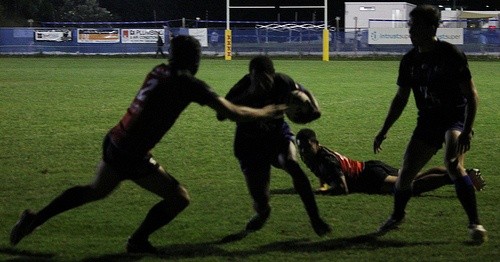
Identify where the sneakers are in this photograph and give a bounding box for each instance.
[465,224,488,243]
[474,169,486,186]
[11,208,37,249]
[378,212,405,236]
[466,169,482,190]
[125,238,167,257]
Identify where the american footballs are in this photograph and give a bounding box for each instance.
[284,91,310,124]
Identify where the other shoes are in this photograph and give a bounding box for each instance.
[246,207,270,233]
[313,219,334,236]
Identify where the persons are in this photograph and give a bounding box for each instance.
[154,32,166,59]
[478,32,487,57]
[218,56,331,237]
[8,34,289,259]
[372,4,488,241]
[295,129,487,197]
